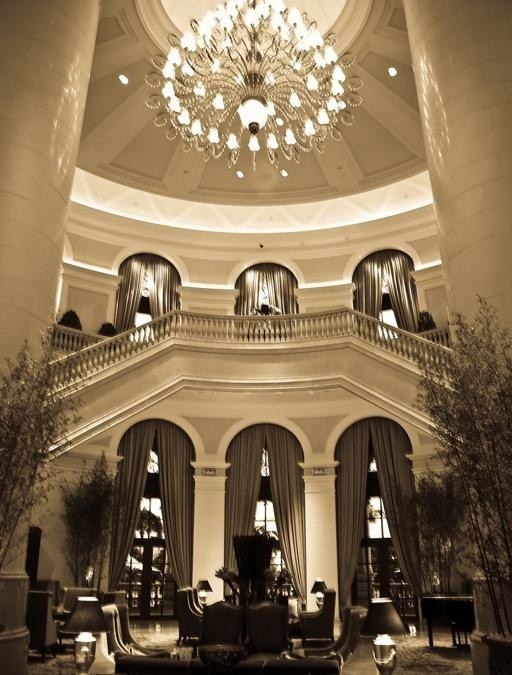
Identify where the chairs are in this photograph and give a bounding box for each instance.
[26,574,474,675]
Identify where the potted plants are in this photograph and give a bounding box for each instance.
[408,297,512,675]
[408,460,474,653]
[1,306,95,675]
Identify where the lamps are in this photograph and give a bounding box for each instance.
[141,0,365,184]
